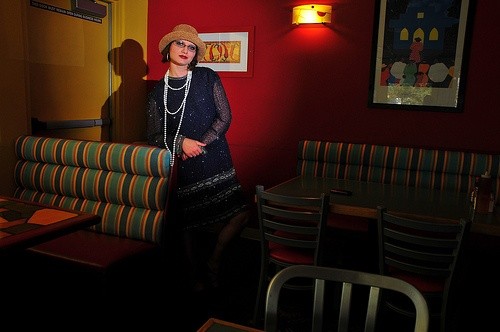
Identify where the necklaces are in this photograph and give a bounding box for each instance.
[163,67,192,166]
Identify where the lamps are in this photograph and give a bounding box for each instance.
[293,4,332,24]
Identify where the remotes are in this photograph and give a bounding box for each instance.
[328,188,352,196]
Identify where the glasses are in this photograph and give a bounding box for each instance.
[173,40,198,52]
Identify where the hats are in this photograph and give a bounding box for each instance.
[159,24,205,62]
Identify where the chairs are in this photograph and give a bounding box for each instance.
[253,185,472,332]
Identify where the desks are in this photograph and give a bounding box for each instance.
[255,174,500,247]
[0,195,101,252]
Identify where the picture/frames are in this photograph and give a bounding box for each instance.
[367,0,477,113]
[194,24,256,78]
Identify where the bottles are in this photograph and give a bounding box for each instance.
[475,171,492,215]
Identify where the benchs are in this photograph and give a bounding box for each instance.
[12,136,174,280]
[284,138,500,273]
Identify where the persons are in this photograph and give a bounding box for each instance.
[144,24,251,292]
